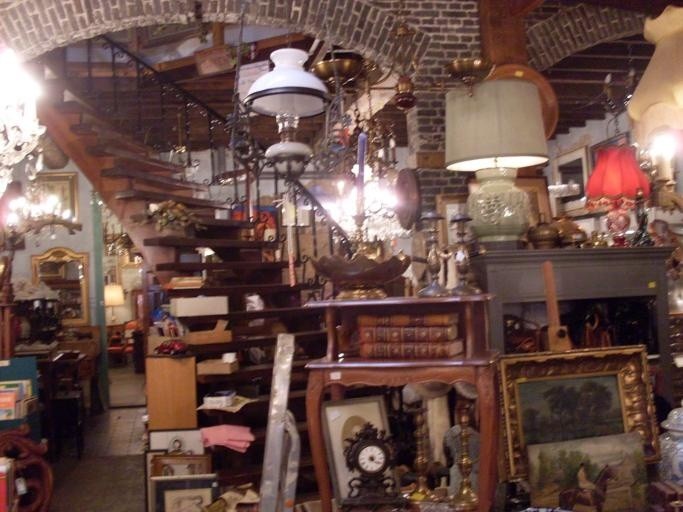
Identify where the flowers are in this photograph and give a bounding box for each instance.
[130,199,208,233]
[127,320,145,333]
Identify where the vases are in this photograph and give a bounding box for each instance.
[132,331,145,374]
[159,222,188,239]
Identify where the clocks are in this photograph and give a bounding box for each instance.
[339,423,409,512]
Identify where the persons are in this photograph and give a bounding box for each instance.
[576,462,603,500]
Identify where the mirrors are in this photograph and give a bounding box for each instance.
[30,248,90,328]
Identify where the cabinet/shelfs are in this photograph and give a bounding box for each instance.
[304,296,501,512]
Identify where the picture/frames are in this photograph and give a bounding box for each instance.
[70,27,138,49]
[144,429,219,512]
[104,233,122,284]
[320,395,399,508]
[119,247,144,268]
[130,289,144,321]
[137,22,213,50]
[29,172,78,227]
[501,345,663,483]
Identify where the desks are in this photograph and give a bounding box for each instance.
[105,321,128,367]
[473,245,676,410]
[34,350,102,463]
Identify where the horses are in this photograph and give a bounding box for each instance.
[559,463,620,512]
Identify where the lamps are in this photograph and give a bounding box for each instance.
[103,282,122,322]
[241,1,683,302]
